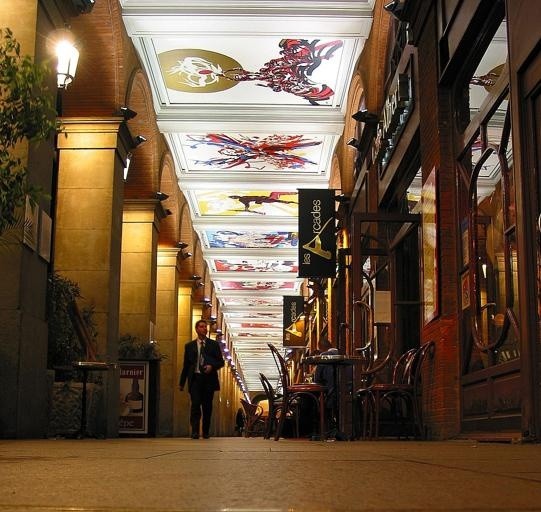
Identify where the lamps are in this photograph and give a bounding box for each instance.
[56,39,81,89]
[133,135,147,147]
[192,272,249,396]
[185,252,192,257]
[154,191,169,201]
[166,208,172,216]
[175,241,189,249]
[121,106,137,121]
[336,108,371,204]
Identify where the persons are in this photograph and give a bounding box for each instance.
[178,320,224,440]
[233,408,244,434]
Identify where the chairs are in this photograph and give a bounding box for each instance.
[241,342,434,440]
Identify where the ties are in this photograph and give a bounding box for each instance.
[199,342,206,372]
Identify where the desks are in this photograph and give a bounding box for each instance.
[51,359,119,439]
